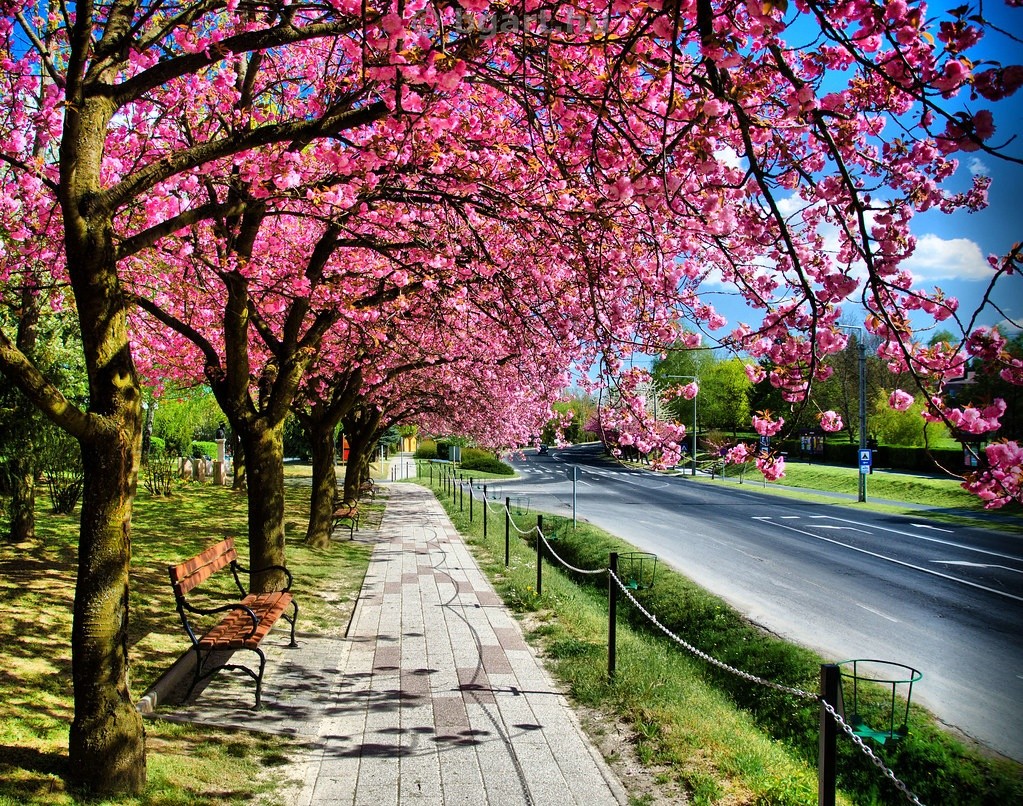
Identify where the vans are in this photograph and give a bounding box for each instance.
[538,444,549,456]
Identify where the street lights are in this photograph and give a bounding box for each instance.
[834,321,866,503]
[667,374,697,476]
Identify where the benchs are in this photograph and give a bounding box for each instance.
[332,496,360,540]
[167,534,299,710]
[359,476,376,500]
